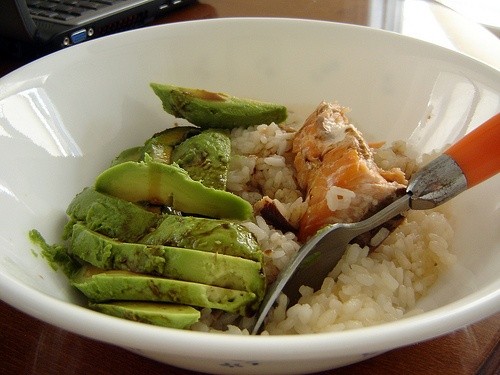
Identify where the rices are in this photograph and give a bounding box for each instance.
[185,102,457,337]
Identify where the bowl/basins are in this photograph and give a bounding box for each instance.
[0,16,500,375]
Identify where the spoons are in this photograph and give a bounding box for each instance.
[254,116,500,335]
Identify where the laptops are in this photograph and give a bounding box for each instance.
[1,0,200,53]
